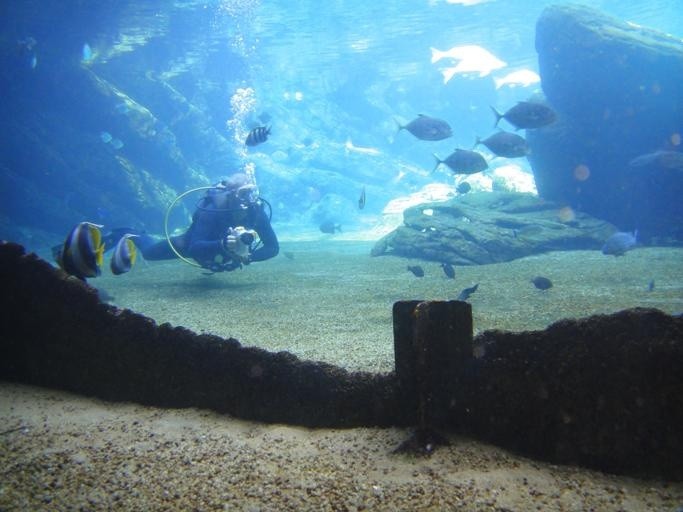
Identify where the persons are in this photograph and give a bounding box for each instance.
[51,172,278,281]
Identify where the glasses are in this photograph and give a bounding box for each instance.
[237,184,259,202]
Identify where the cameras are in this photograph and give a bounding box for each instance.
[233,227,258,257]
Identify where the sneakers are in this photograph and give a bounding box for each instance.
[102,232,123,249]
